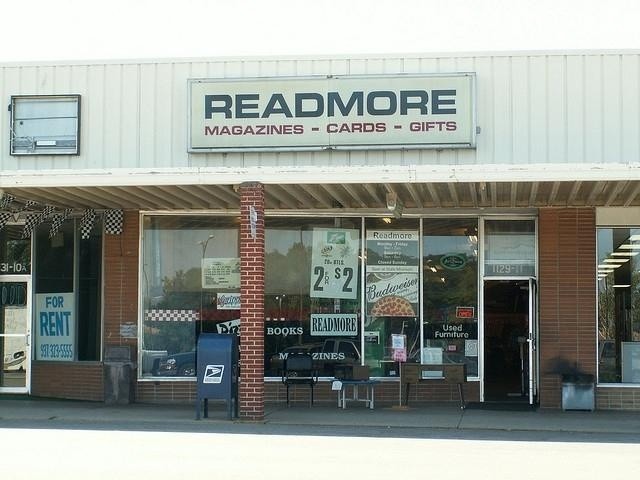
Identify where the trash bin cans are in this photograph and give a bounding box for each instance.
[562,374,595,413]
[102,346,136,405]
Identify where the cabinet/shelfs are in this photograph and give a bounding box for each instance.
[401,361,468,411]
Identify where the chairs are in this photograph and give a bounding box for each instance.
[282,350,320,407]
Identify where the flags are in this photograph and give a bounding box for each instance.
[0,194,16,209]
[0,212,12,231]
[104,209,123,235]
[41,205,55,223]
[62,208,73,219]
[80,214,96,240]
[79,208,95,229]
[21,200,37,212]
[20,214,42,240]
[49,214,65,241]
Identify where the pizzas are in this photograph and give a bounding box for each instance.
[371,295,416,315]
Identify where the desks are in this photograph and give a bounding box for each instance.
[333,380,382,411]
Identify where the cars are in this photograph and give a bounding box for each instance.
[152,352,195,377]
[270,338,361,368]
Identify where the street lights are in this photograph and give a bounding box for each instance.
[275,294,286,313]
[198,235,215,333]
